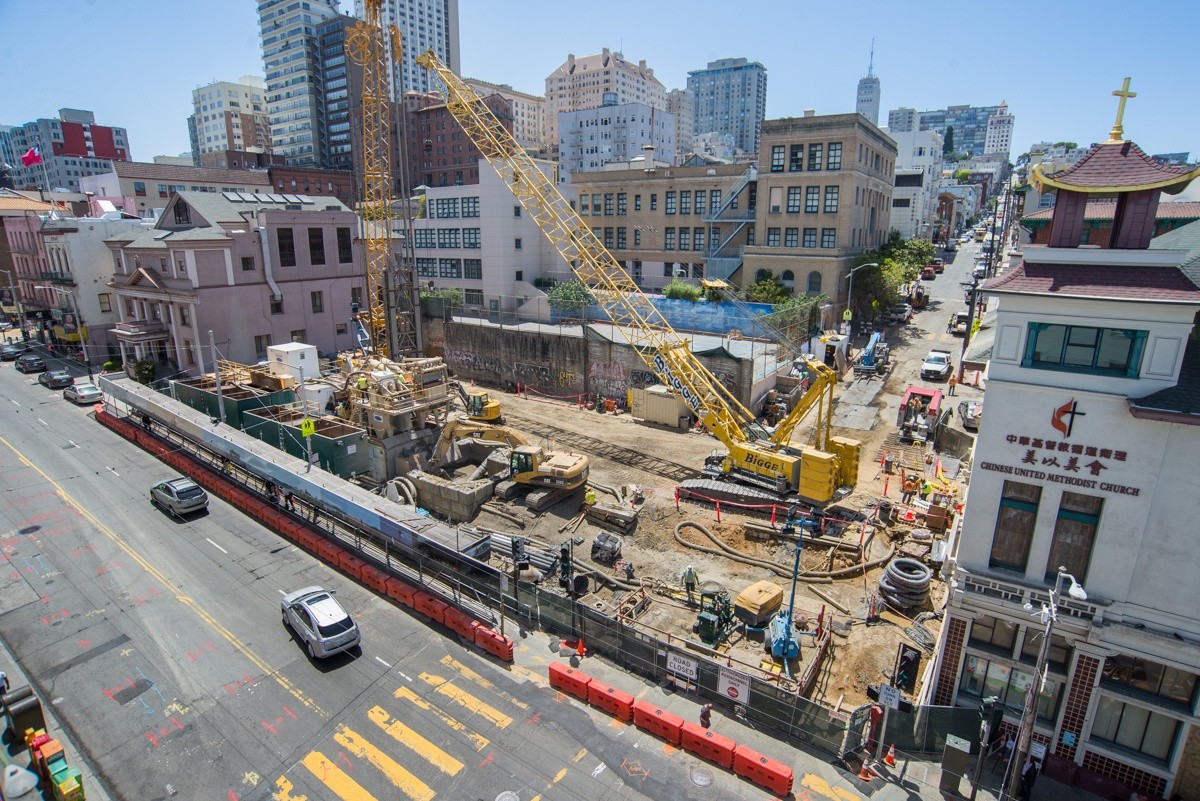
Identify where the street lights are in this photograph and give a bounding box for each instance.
[34,285,95,384]
[1005,566,1088,800]
[910,223,930,239]
[258,359,314,465]
[945,199,961,228]
[845,263,879,326]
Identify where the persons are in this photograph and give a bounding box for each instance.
[62,314,76,331]
[909,395,922,423]
[325,371,405,424]
[48,344,85,364]
[699,703,713,729]
[948,375,957,395]
[681,566,699,601]
[902,475,916,505]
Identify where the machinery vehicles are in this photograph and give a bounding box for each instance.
[973,220,992,242]
[415,47,862,513]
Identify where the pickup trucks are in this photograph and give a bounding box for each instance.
[889,302,913,322]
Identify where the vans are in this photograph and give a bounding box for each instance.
[982,218,1003,250]
[965,282,981,304]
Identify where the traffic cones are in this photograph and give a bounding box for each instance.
[884,743,897,766]
[859,758,872,781]
[574,639,586,656]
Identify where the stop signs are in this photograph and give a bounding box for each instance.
[727,687,739,698]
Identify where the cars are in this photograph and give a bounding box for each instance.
[973,260,988,278]
[952,312,970,333]
[0,344,104,405]
[280,585,361,661]
[946,224,982,252]
[150,477,209,518]
[921,257,945,279]
[920,349,952,379]
[957,398,983,429]
